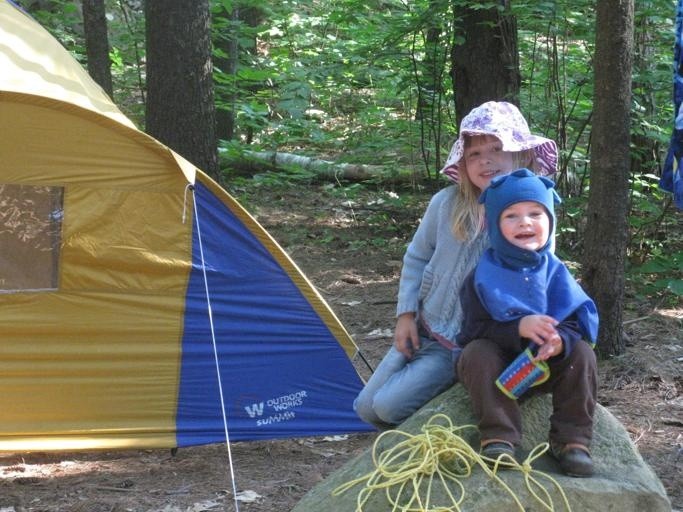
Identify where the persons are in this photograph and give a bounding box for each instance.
[454,167,601,480]
[351,99,559,434]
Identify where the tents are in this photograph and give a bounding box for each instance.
[0,0,377,456]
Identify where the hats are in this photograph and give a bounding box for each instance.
[435,98,559,192]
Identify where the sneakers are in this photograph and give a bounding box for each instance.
[477,441,518,471]
[546,435,595,480]
